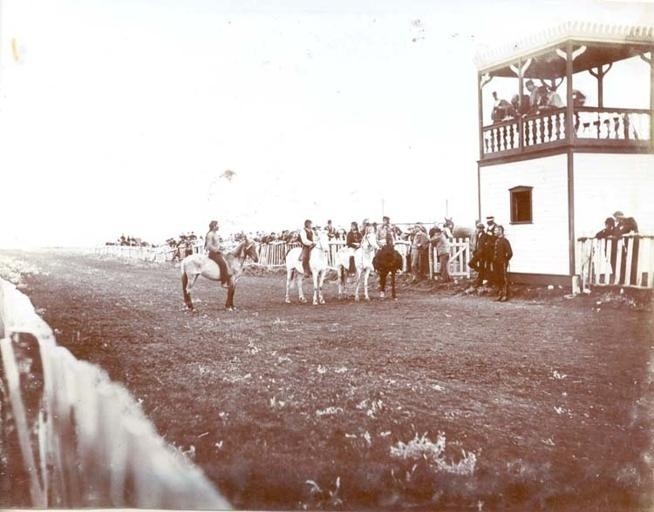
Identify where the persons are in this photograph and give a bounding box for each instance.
[247,221,402,242]
[300,220,318,278]
[205,222,229,290]
[490,77,587,145]
[167,231,198,262]
[611,211,641,284]
[219,232,246,242]
[377,217,395,248]
[347,223,363,278]
[595,216,622,276]
[402,223,453,283]
[465,216,515,302]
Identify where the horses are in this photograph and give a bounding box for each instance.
[180,235,258,313]
[372,230,403,301]
[284,229,330,305]
[335,229,379,302]
